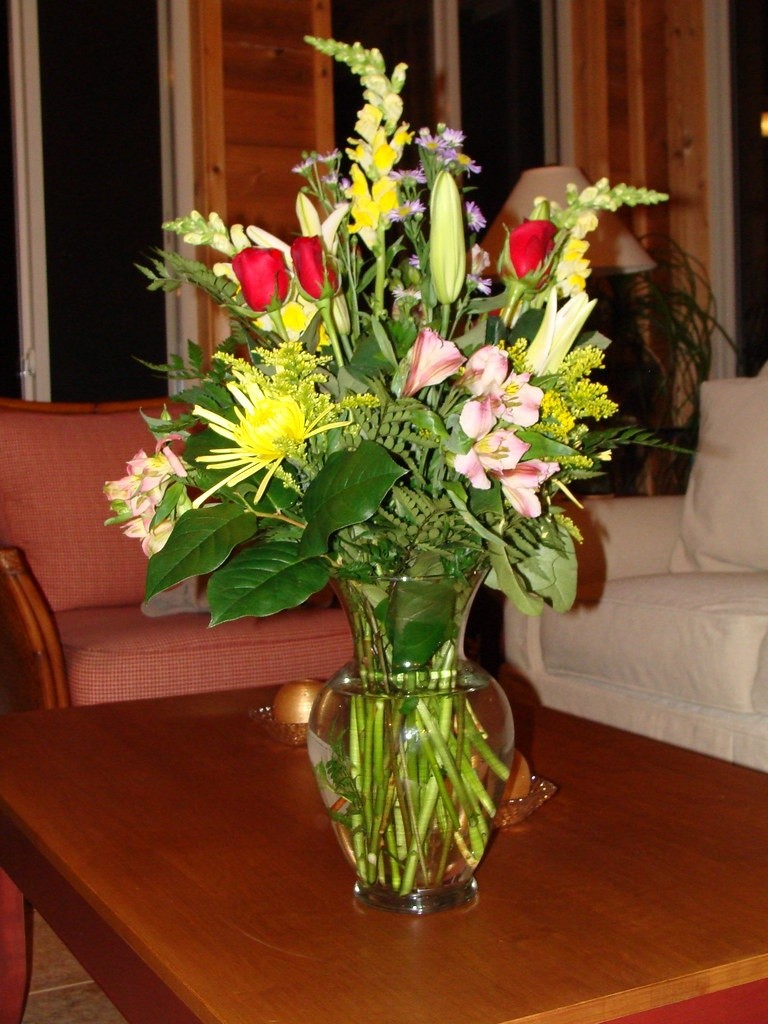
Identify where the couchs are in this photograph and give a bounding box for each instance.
[504,360,768,771]
[0,399,356,1024]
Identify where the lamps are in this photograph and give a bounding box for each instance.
[464,164,658,281]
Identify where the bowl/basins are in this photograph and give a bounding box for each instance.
[488,777,556,829]
[248,706,308,746]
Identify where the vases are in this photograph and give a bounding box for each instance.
[307,559,517,917]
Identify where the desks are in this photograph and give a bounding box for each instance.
[0,680,768,1024]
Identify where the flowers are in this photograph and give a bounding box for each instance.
[100,34,671,628]
